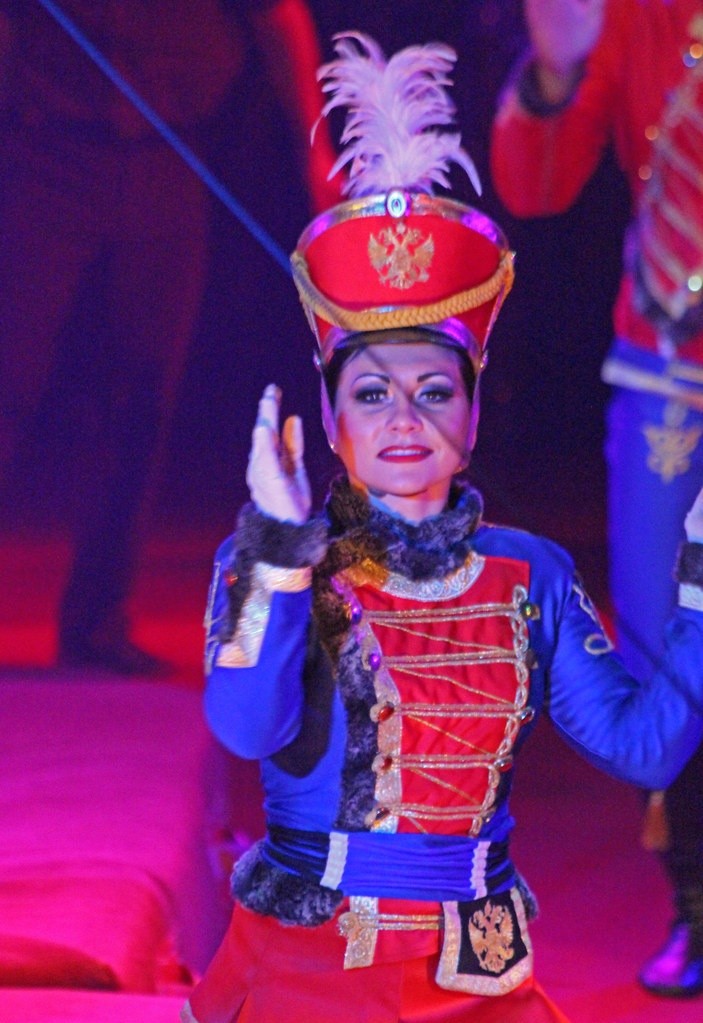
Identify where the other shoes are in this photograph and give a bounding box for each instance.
[635,887,702,997]
[63,634,169,679]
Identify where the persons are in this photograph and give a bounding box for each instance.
[180,187,703,1022]
[1,0,352,681]
[482,0,702,995]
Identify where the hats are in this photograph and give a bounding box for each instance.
[290,32,515,371]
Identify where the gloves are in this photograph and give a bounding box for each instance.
[522,1,603,72]
[244,383,311,523]
